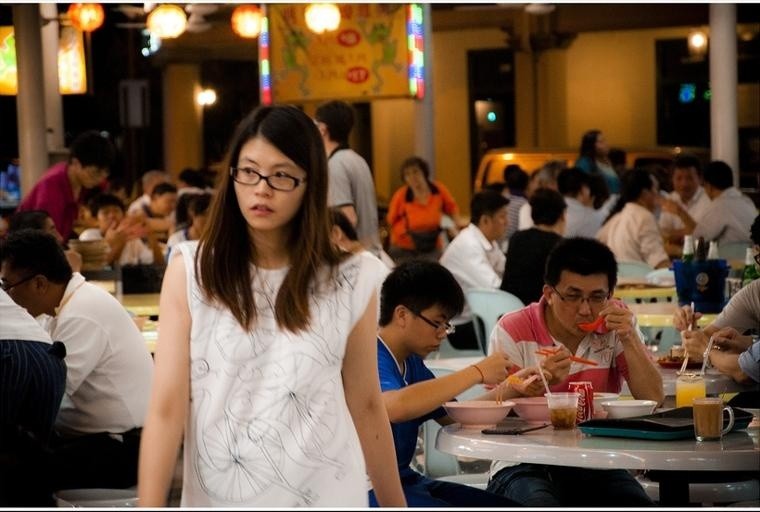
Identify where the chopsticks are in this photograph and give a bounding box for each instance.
[490,335,505,406]
[701,336,714,372]
[535,347,600,368]
[680,352,690,374]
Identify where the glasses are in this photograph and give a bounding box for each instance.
[401,304,457,335]
[551,285,611,306]
[228,166,308,192]
[0,276,35,292]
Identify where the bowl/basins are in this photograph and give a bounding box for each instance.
[67,238,110,269]
[442,390,658,430]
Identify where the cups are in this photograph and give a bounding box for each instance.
[675,376,706,411]
[691,438,727,471]
[690,396,735,441]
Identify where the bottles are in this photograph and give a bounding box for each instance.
[741,245,759,291]
[681,234,721,263]
[723,269,741,310]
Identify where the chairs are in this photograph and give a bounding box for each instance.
[631,472,759,509]
[613,286,679,305]
[712,240,753,262]
[617,259,654,282]
[462,287,527,361]
[433,310,484,357]
[421,364,497,493]
[621,302,720,328]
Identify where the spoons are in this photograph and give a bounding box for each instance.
[575,314,606,332]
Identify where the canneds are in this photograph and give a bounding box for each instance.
[567,381,593,423]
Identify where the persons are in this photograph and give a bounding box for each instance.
[0,103,759,508]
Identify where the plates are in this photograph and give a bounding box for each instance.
[657,357,704,371]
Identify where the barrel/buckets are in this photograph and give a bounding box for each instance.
[668,259,731,314]
